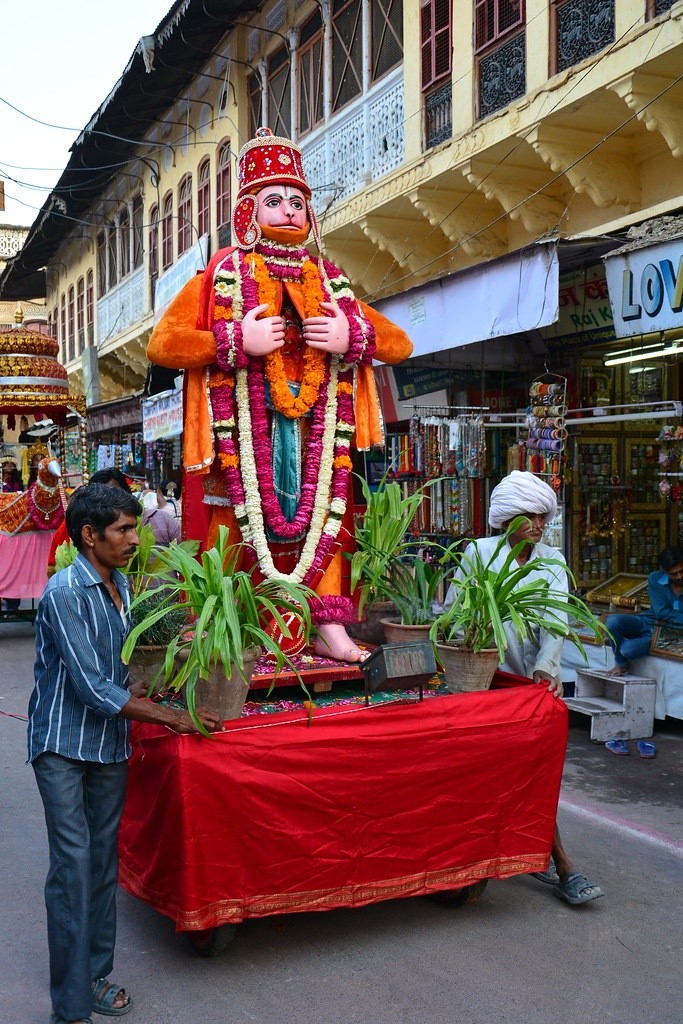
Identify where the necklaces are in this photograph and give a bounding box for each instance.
[203,243,361,606]
[390,413,485,539]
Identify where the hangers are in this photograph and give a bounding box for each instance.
[527,361,567,477]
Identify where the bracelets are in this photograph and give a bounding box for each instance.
[524,383,569,451]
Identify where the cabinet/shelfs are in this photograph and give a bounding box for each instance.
[564,357,683,662]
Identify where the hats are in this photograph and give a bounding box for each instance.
[487,469,558,528]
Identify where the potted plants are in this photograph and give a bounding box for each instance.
[342,443,618,692]
[53,513,332,738]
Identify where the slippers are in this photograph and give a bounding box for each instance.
[532,860,560,884]
[89,977,134,1016]
[48,1010,93,1024]
[605,739,629,755]
[553,872,604,904]
[637,741,657,758]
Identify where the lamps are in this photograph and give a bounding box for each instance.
[360,640,438,707]
[601,338,683,368]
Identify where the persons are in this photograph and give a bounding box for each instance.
[0,455,23,494]
[46,469,183,604]
[145,139,414,665]
[605,545,683,676]
[443,470,605,904]
[23,453,45,491]
[26,484,225,1024]
